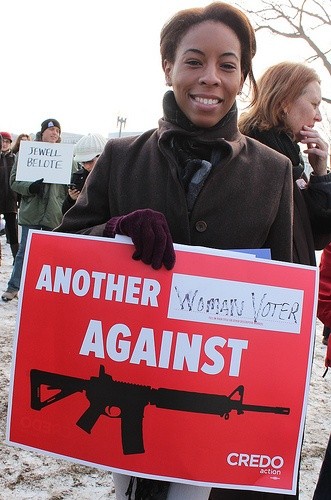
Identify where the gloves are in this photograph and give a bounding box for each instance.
[102,209,176,271]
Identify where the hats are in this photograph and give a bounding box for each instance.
[73,133,107,162]
[0,131,13,143]
[41,119,61,136]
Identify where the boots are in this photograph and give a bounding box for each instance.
[9,243,20,266]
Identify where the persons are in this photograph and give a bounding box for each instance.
[61,134,107,215]
[311,242,331,500]
[239,60,331,251]
[1,119,79,301]
[0,131,20,265]
[11,134,30,153]
[51,0,294,500]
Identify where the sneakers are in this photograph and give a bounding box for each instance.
[2,286,19,301]
